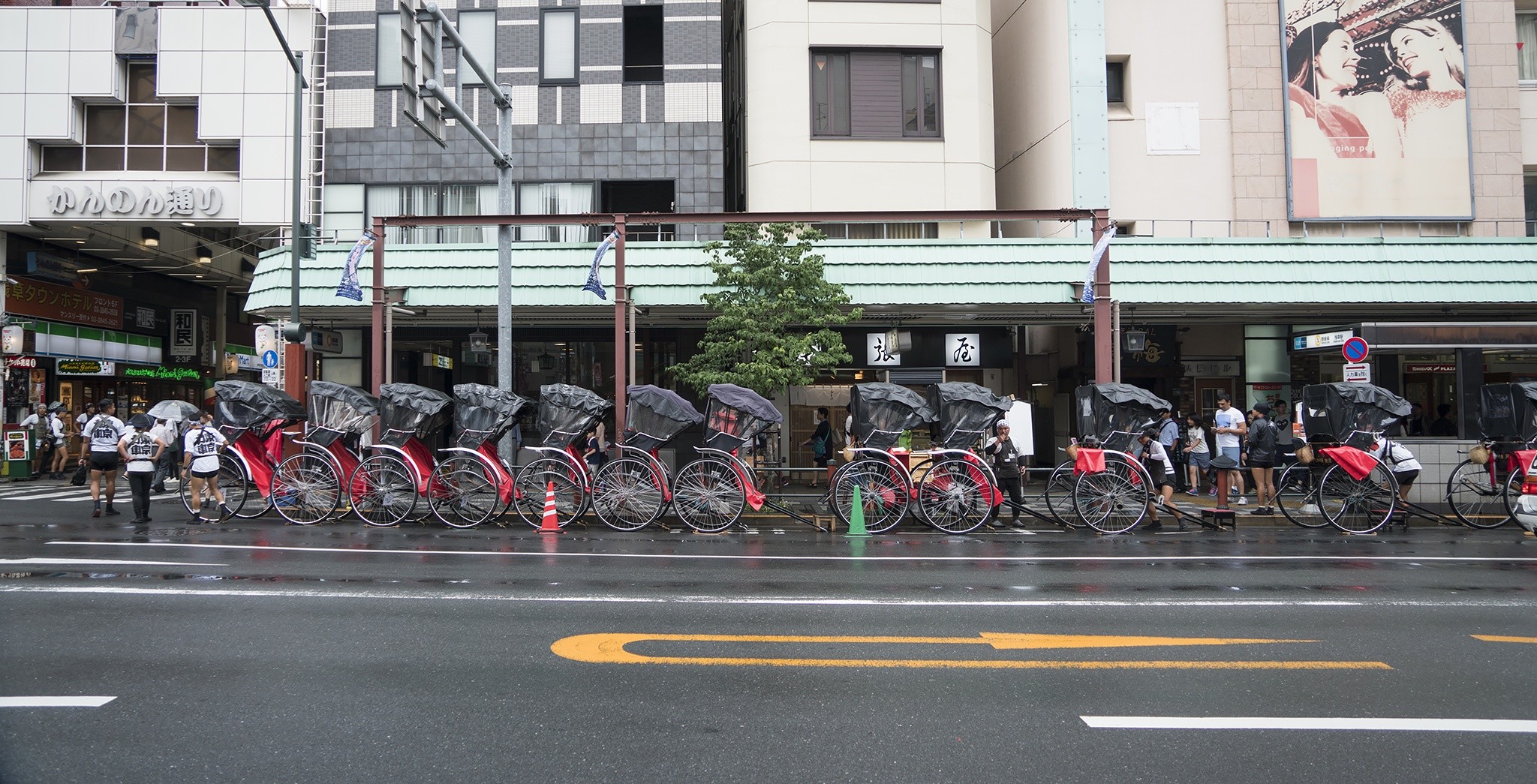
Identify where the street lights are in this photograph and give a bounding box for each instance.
[235,1,310,485]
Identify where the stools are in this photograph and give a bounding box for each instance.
[1367,510,1407,534]
[813,513,837,532]
[1200,509,1235,531]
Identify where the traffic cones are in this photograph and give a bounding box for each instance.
[845,484,874,537]
[537,481,563,534]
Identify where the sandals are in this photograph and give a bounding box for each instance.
[806,482,817,488]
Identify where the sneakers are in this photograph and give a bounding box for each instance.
[1185,484,1303,515]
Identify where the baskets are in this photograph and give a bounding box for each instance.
[1066,443,1083,461]
[1469,445,1490,464]
[1294,444,1314,464]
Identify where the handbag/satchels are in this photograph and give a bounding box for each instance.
[811,441,824,454]
[45,434,57,443]
[71,466,86,486]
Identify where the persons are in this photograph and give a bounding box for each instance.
[1137,429,1188,530]
[20,398,229,524]
[1400,403,1424,437]
[581,418,606,507]
[1182,411,1218,496]
[798,402,890,489]
[1211,393,1248,505]
[1241,399,1307,515]
[338,408,373,508]
[1125,408,1188,499]
[513,421,522,451]
[348,246,362,285]
[1225,433,1245,503]
[985,419,1026,527]
[1287,15,1468,159]
[1429,403,1453,437]
[1367,433,1422,529]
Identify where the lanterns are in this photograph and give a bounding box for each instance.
[226,353,238,374]
[255,324,275,361]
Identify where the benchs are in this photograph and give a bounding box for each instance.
[713,447,739,491]
[563,443,591,488]
[641,445,663,491]
[888,447,919,499]
[307,434,338,490]
[214,421,248,478]
[473,445,491,487]
[390,435,427,487]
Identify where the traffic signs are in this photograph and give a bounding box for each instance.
[1343,362,1371,383]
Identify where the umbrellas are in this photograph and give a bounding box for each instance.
[146,400,200,421]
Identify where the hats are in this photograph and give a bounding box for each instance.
[1253,401,1270,414]
[47,401,64,410]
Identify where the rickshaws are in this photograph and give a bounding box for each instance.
[903,382,1076,536]
[1035,382,1227,535]
[1442,381,1537,537]
[1276,381,1470,534]
[270,380,431,528]
[816,382,996,535]
[589,385,749,533]
[671,383,829,534]
[348,382,515,531]
[174,379,344,525]
[426,382,590,530]
[511,383,672,532]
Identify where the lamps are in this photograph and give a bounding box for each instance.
[468,309,489,354]
[241,257,255,278]
[538,342,554,368]
[1122,306,1147,351]
[197,242,213,263]
[143,225,160,248]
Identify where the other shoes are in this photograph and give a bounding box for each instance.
[1142,523,1163,529]
[143,516,152,521]
[1178,518,1188,529]
[154,485,162,493]
[220,510,229,522]
[1012,519,1026,527]
[774,479,789,488]
[168,477,180,482]
[92,508,100,517]
[203,503,209,507]
[164,478,171,483]
[130,518,142,522]
[50,472,67,481]
[105,507,120,514]
[32,472,43,478]
[186,517,200,524]
[992,520,1006,527]
[121,475,128,480]
